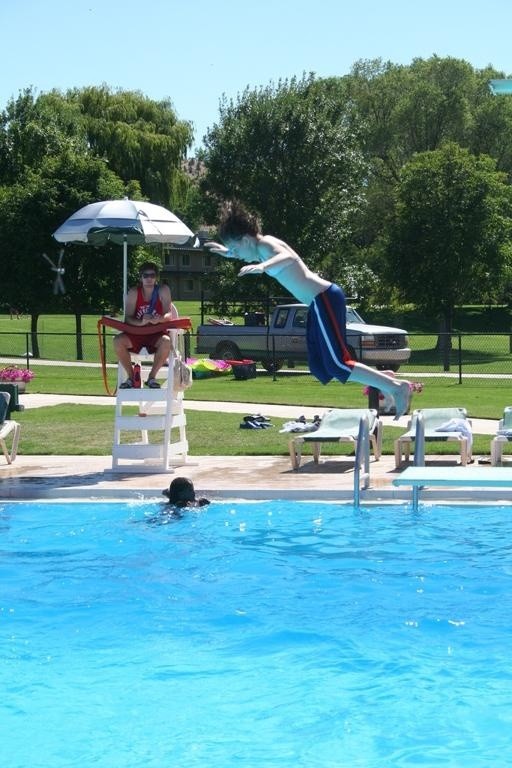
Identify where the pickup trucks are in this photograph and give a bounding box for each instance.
[194,303,412,373]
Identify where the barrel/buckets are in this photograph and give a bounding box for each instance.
[245,312,256,325]
[256,309,265,326]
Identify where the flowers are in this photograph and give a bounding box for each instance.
[0,365,35,385]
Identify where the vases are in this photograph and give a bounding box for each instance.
[1,379,26,394]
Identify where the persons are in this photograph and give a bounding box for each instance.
[204,207,410,422]
[151,478,211,523]
[112,262,173,388]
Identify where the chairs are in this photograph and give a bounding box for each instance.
[286,407,511,474]
[104,303,198,477]
[1,382,25,465]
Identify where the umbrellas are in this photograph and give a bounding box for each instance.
[50,195,200,321]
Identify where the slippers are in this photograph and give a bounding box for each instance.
[119,378,134,389]
[144,378,161,388]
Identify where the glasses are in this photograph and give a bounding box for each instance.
[142,273,156,278]
